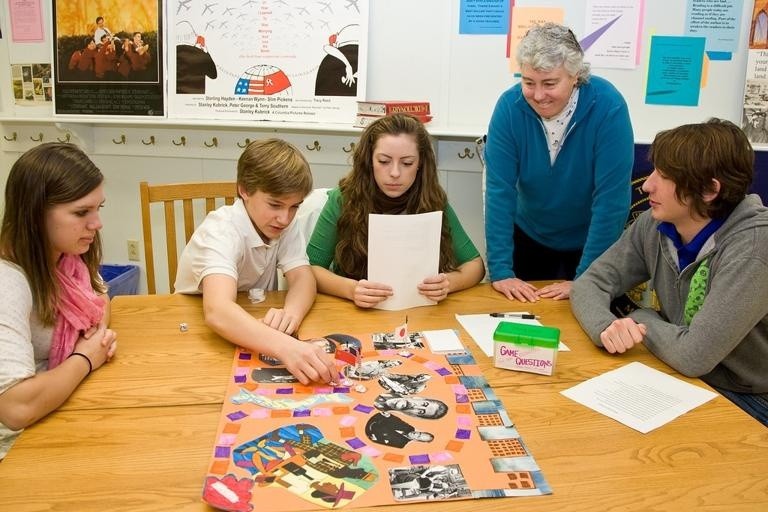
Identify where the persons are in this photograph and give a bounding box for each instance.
[306,114,486,313]
[0,142,119,464]
[174,136,342,387]
[63,15,153,82]
[301,324,464,503]
[481,22,635,303]
[567,119,767,428]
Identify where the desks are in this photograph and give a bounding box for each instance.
[0,280,767,512]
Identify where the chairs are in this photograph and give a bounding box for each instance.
[139,180,237,294]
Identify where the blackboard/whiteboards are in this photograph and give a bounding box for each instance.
[0,0,755,147]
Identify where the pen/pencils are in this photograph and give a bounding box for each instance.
[489,313,541,319]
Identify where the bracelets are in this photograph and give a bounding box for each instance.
[67,353,92,377]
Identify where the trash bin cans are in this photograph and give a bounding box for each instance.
[97,263,140,301]
